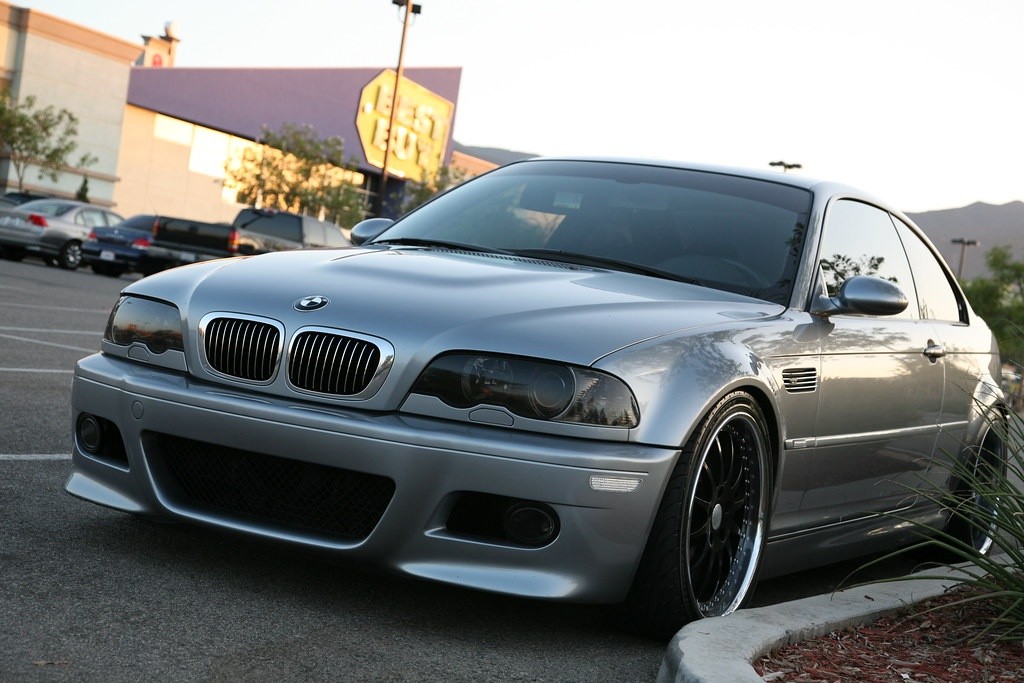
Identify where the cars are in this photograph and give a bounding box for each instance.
[0,190,54,219]
[80,211,162,277]
[0,198,129,271]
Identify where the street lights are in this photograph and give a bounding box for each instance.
[374,0,423,218]
[950,237,982,283]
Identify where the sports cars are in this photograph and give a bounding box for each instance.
[63,157,1016,639]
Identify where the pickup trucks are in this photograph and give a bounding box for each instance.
[139,204,355,280]
